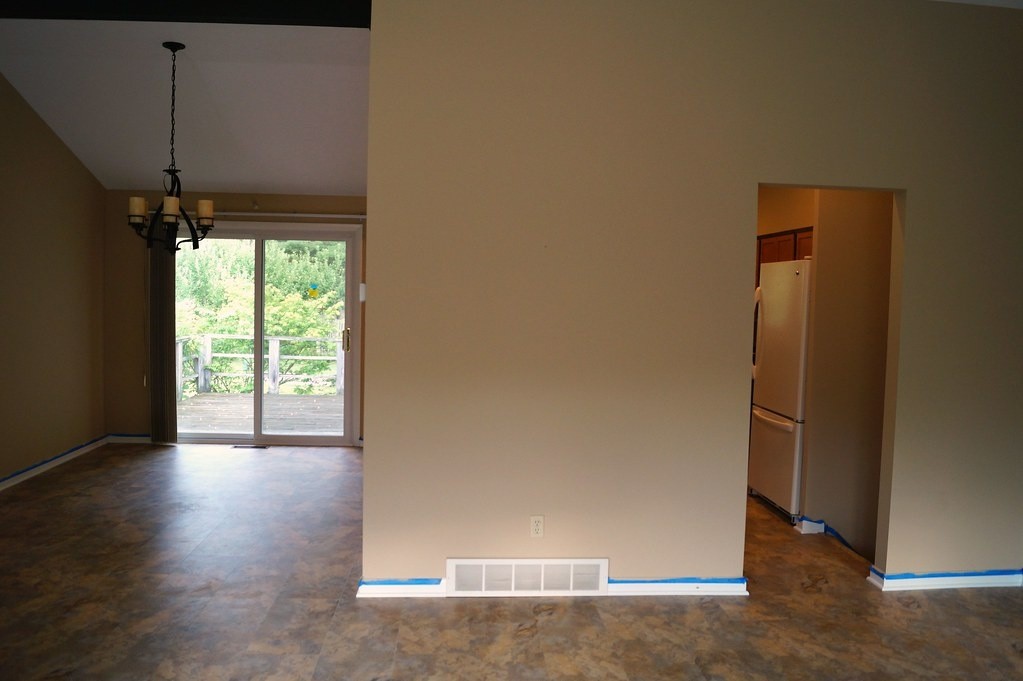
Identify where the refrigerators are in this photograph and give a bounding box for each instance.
[747,255,812,525]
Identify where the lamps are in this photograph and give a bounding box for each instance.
[126,41,215,256]
